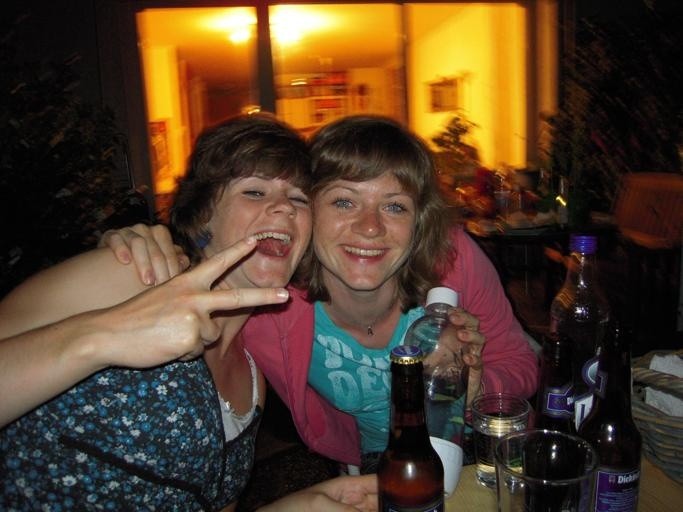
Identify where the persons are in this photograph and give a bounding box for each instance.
[99,116,539,475]
[0,113,378,508]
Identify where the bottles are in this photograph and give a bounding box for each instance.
[574,322,642,511]
[522,334,577,511]
[377,344,445,511]
[403,287,470,498]
[550,235,608,432]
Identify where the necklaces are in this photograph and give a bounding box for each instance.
[335,304,396,336]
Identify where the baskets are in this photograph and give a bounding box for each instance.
[631,351,683,484]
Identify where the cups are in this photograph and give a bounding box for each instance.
[470,393,530,490]
[429,436,464,499]
[448,201,469,227]
[492,427,601,512]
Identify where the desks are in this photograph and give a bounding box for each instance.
[445,463,683,512]
[592,209,682,307]
[462,187,579,305]
